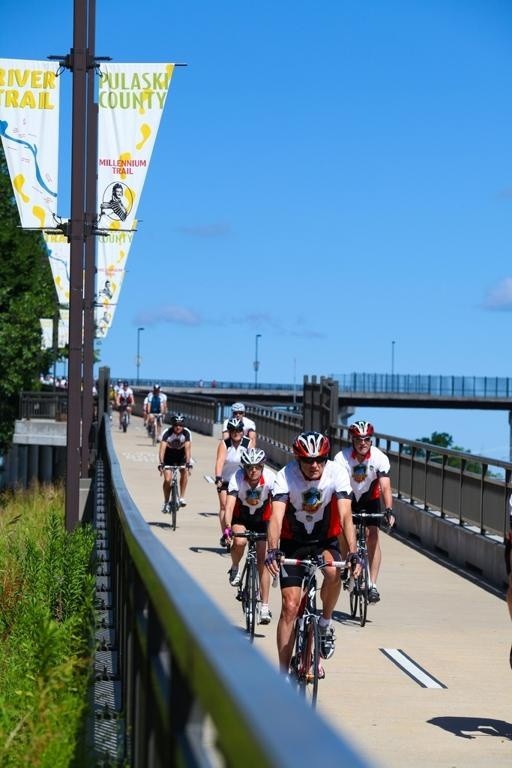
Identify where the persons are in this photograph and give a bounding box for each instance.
[39,374,168,442]
[505,492,512,669]
[216,418,252,546]
[264,432,367,676]
[225,448,276,622]
[222,403,256,448]
[158,413,192,513]
[334,421,395,603]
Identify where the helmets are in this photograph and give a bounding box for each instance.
[154,384,161,389]
[292,430,330,457]
[241,448,266,466]
[348,420,374,439]
[117,379,127,385]
[170,413,186,425]
[227,417,244,428]
[231,403,246,412]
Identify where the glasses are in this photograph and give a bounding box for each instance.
[229,427,244,432]
[298,456,326,463]
[247,463,263,470]
[351,435,370,443]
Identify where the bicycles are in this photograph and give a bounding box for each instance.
[220,529,271,638]
[160,465,191,530]
[341,506,396,627]
[275,551,360,712]
[145,412,166,446]
[116,403,133,433]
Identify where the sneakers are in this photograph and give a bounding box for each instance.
[221,534,226,546]
[341,558,348,580]
[179,498,186,507]
[317,618,335,660]
[369,588,380,603]
[162,503,169,513]
[229,568,241,586]
[260,612,272,622]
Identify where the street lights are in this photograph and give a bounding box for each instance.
[136,327,145,384]
[391,340,397,392]
[252,333,263,388]
